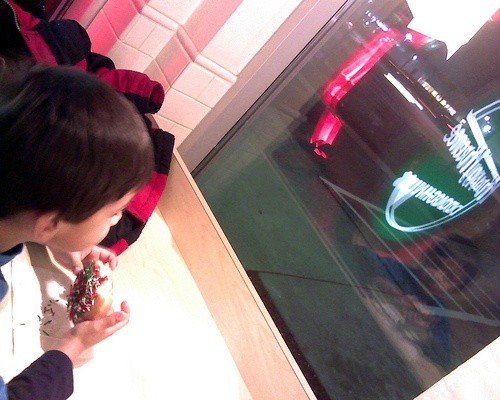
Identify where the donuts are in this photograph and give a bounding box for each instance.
[65,260,114,326]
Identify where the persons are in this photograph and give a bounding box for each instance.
[338,219,487,353]
[0,65,155,400]
